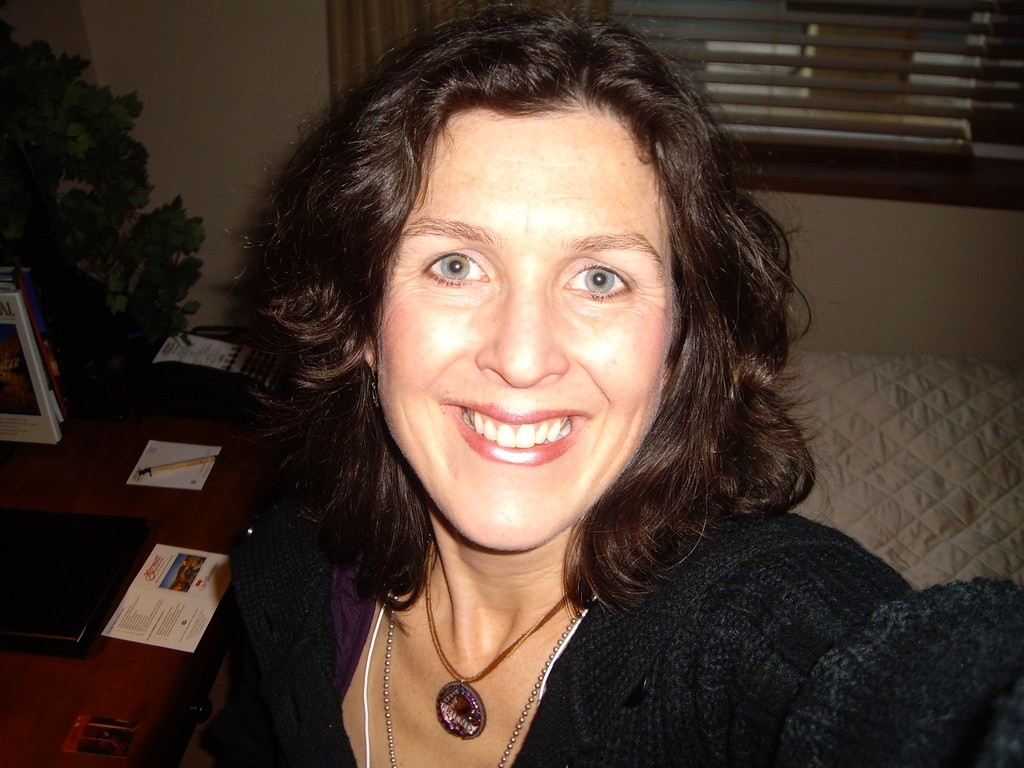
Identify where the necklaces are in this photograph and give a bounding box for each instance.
[427,538,570,741]
[383,593,583,767]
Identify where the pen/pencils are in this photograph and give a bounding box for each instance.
[137,456,219,475]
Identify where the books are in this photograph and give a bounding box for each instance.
[1,506,152,659]
[0,264,69,445]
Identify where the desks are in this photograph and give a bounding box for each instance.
[1,414,279,768]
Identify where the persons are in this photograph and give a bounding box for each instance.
[203,5,1024,767]
